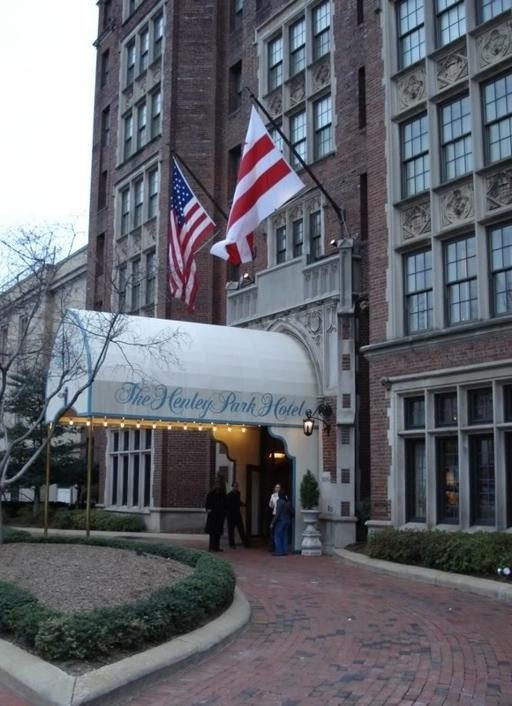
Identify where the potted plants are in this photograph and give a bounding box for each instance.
[300,470,322,557]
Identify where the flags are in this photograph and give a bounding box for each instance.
[210,100,306,267]
[167,154,217,312]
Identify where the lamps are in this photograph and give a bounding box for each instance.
[303,404,332,436]
[330,240,338,253]
[243,272,253,281]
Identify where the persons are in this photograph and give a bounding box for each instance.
[226,481,249,550]
[268,482,288,539]
[204,474,228,553]
[268,490,295,555]
